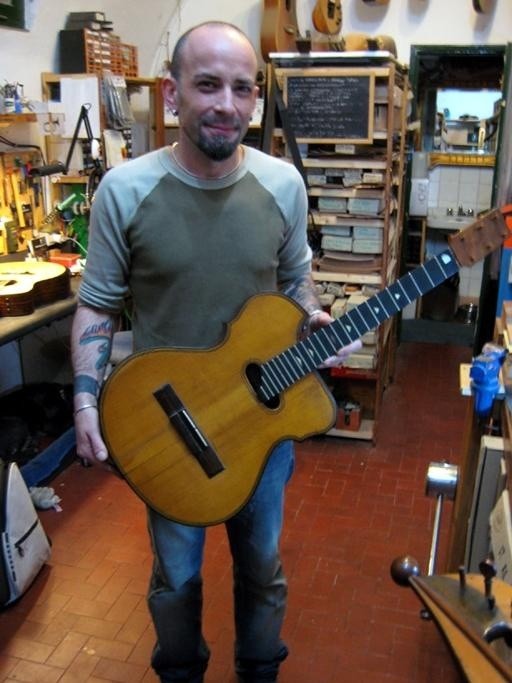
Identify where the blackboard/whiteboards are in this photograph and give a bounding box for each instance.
[282,68,375,145]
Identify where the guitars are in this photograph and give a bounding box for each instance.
[0,262,71,318]
[261,1,300,63]
[96,211,508,526]
[312,1,342,35]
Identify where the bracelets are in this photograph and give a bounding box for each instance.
[73,404,98,417]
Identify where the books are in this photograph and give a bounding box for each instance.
[304,165,397,371]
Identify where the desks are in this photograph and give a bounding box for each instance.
[1,242,83,489]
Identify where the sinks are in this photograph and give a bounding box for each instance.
[427,210,473,229]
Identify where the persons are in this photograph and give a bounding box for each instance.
[70,21,364,682]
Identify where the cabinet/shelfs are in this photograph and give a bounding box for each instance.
[1,110,66,180]
[43,52,410,443]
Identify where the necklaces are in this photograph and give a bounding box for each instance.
[170,141,243,182]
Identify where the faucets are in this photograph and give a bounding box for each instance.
[457,204,465,215]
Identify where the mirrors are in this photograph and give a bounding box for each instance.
[414,84,502,152]
